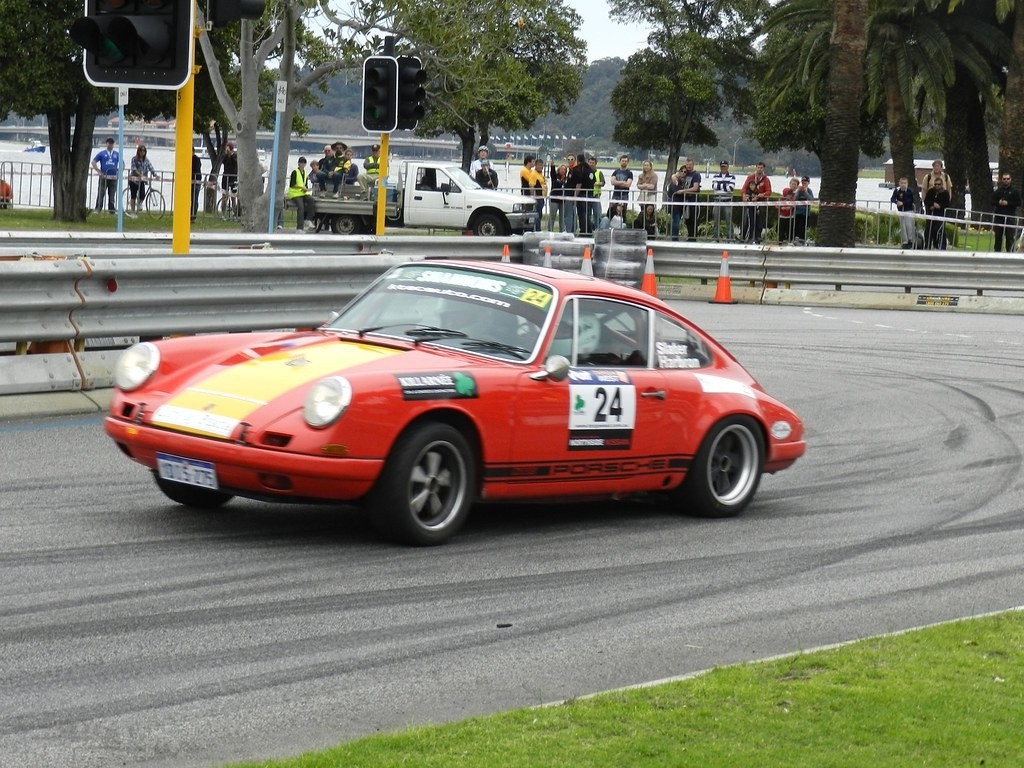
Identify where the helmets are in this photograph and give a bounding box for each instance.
[547,311,601,363]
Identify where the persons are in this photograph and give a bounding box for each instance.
[129,145,160,214]
[992,172,1021,253]
[741,161,814,246]
[191,148,202,222]
[711,161,736,244]
[547,310,602,366]
[669,159,702,241]
[469,145,494,179]
[607,203,626,224]
[476,160,498,190]
[91,138,124,215]
[221,143,238,221]
[890,160,953,250]
[357,144,389,201]
[287,156,316,234]
[638,204,657,239]
[548,153,606,233]
[308,142,359,199]
[637,160,658,210]
[610,155,633,206]
[520,156,548,232]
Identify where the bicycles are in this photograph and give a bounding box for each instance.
[216,181,241,222]
[122,178,166,221]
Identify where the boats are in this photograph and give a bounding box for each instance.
[24,145,46,153]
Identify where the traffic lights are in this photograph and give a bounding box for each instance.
[361,55,399,134]
[395,55,427,132]
[205,0,266,32]
[70,0,194,89]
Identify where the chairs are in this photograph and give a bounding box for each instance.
[588,340,643,366]
[422,176,436,191]
[440,305,521,347]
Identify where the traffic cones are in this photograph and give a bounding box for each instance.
[542,245,552,269]
[579,247,594,277]
[640,248,658,299]
[500,244,511,263]
[709,250,739,305]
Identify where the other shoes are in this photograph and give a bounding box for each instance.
[304,219,315,228]
[277,225,282,229]
[131,214,138,218]
[332,192,338,198]
[138,204,142,211]
[296,229,306,234]
[320,190,327,198]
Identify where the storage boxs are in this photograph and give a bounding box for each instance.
[374,183,397,202]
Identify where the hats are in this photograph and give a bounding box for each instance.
[720,161,729,166]
[371,144,380,150]
[331,141,347,151]
[802,176,810,182]
[478,146,489,154]
[106,138,114,144]
[481,159,490,165]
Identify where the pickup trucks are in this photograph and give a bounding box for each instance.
[311,159,540,236]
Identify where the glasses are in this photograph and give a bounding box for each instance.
[680,170,686,173]
[1003,178,1010,180]
[568,159,573,161]
[934,184,940,186]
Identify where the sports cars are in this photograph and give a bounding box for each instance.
[103,258,807,549]
